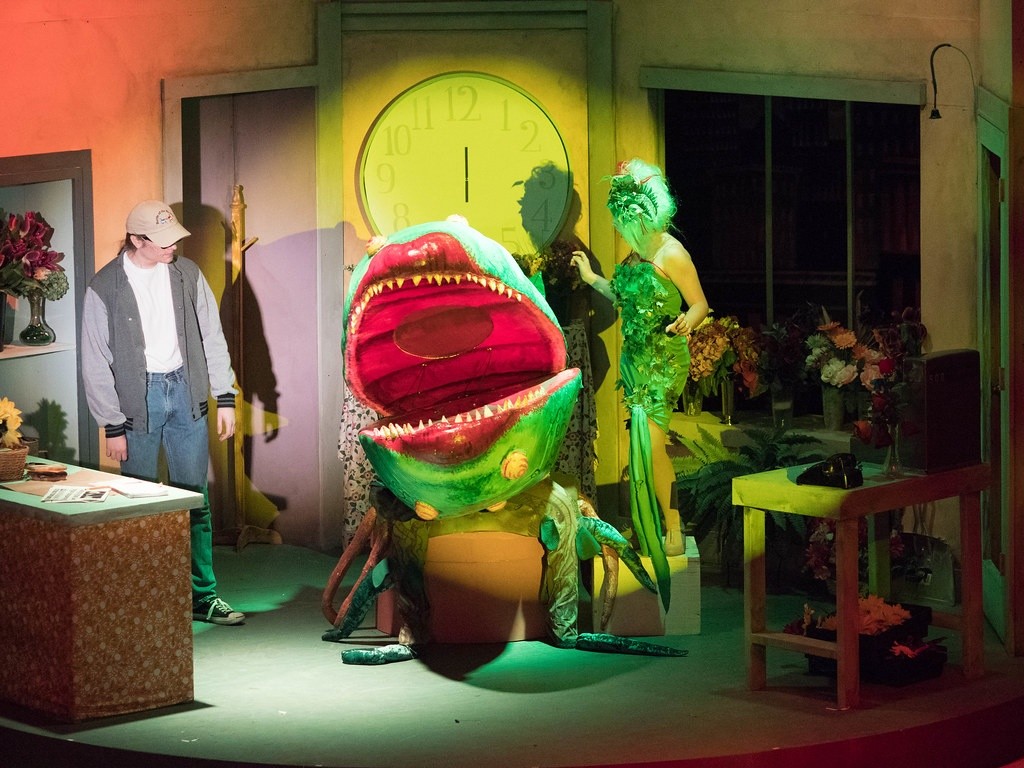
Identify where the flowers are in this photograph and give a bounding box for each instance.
[762,308,807,390]
[783,601,814,635]
[807,319,863,384]
[0,210,70,300]
[694,308,714,330]
[851,322,924,441]
[687,321,762,385]
[0,397,24,449]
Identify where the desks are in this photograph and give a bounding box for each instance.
[730,453,984,712]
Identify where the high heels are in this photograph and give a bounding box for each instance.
[663,526,687,556]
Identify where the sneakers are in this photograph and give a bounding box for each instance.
[192,597,245,624]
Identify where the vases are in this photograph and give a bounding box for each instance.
[0,292,7,352]
[818,387,845,432]
[0,447,29,481]
[684,381,703,417]
[18,297,56,343]
[718,387,737,425]
[881,420,903,478]
[773,381,793,431]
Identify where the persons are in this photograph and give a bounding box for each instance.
[567,159,709,556]
[81,201,244,624]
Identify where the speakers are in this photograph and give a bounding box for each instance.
[901,349,981,473]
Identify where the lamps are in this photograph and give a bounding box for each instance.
[928,41,974,118]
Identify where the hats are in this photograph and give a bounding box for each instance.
[126,200,192,248]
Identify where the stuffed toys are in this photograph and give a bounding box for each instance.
[320,213,690,665]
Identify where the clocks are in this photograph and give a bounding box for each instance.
[354,71,573,260]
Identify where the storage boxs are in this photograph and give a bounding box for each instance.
[593,534,701,635]
[803,600,948,686]
